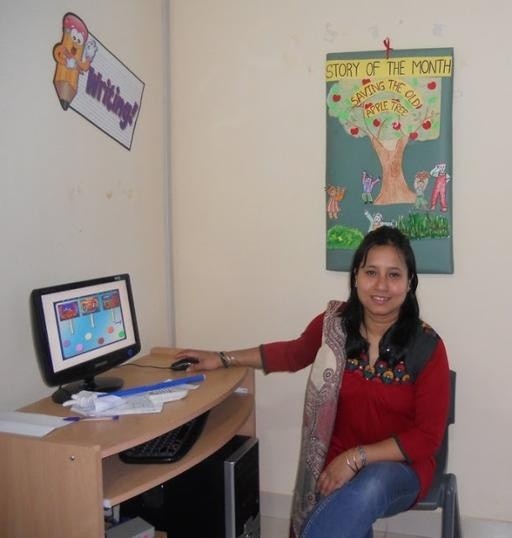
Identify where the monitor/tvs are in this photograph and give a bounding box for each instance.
[29,273,141,404]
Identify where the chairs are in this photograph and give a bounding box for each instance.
[410,370,462,538]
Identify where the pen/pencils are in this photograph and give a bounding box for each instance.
[63,416,119,421]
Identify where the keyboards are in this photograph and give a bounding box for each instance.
[118,409,210,463]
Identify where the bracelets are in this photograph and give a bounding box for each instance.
[358,443,367,467]
[216,350,240,368]
[344,450,358,473]
[350,447,359,472]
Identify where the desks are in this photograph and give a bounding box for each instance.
[0,346,256,538]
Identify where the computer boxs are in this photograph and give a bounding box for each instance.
[118,434,261,537]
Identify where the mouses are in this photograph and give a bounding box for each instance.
[171,358,200,371]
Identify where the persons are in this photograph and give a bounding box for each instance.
[173,223,454,537]
